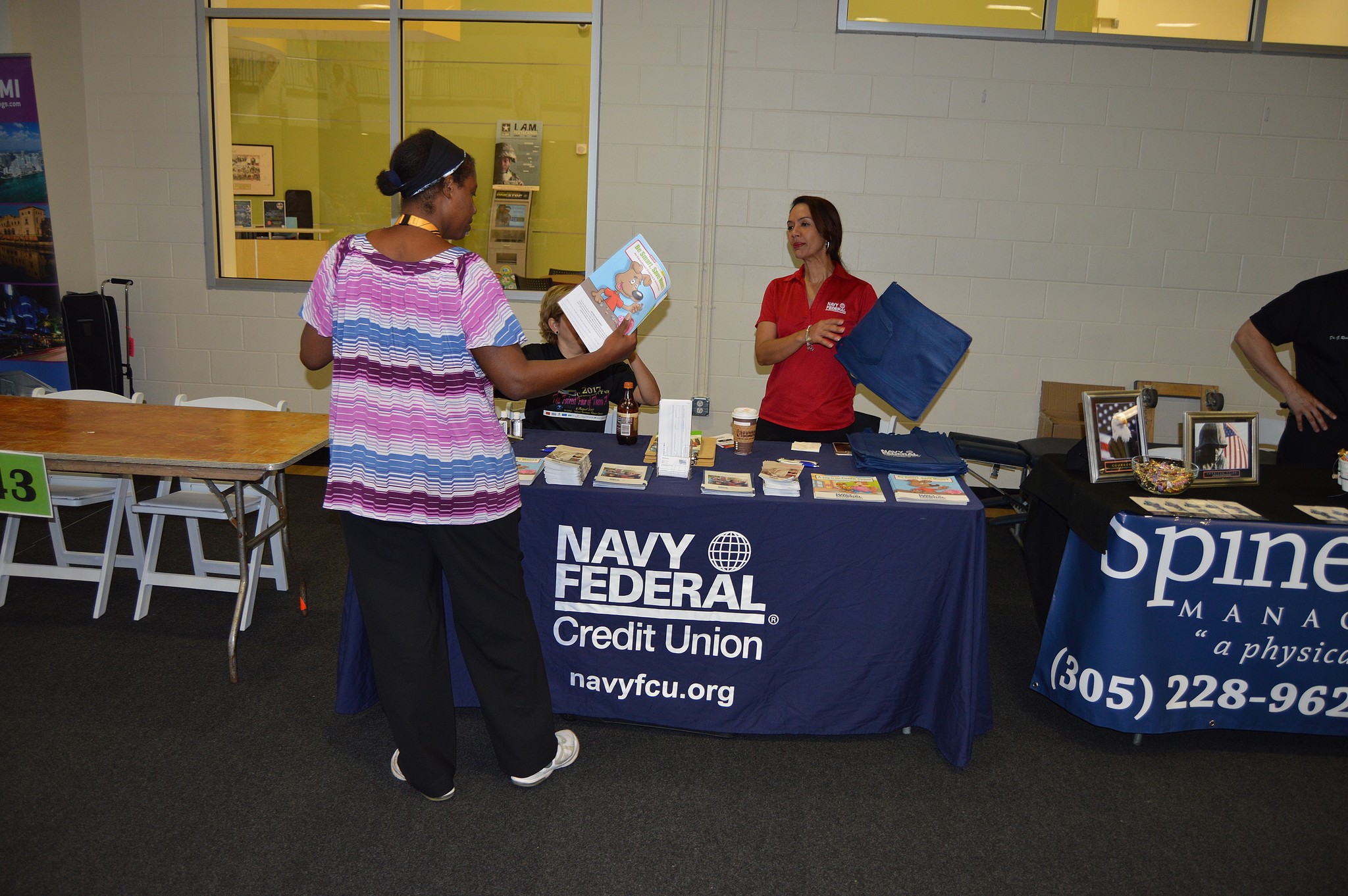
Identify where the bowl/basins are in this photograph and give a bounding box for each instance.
[1131,456,1199,496]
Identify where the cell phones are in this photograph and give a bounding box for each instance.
[833,442,852,454]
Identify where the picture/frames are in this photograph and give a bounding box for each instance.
[231,144,275,196]
[1183,411,1260,488]
[1081,390,1148,483]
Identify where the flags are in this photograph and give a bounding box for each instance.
[1223,422,1248,469]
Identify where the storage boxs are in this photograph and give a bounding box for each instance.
[1037,380,1125,439]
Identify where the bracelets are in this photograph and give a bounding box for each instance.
[805,324,814,351]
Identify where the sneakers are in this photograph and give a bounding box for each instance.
[511,730,580,787]
[391,748,455,801]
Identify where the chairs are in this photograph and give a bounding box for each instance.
[514,273,554,291]
[0,387,146,619]
[853,411,897,436]
[131,395,288,631]
[548,268,585,274]
[506,402,618,434]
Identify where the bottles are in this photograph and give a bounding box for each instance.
[616,382,639,445]
[498,410,511,435]
[511,412,523,438]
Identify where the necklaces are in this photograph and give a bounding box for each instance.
[393,214,441,238]
[804,264,835,309]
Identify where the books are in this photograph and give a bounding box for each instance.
[700,469,756,497]
[758,460,804,497]
[643,430,717,467]
[811,473,886,503]
[542,445,593,487]
[514,457,545,486]
[592,461,654,490]
[888,473,970,506]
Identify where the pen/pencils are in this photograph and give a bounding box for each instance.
[545,445,558,447]
[539,447,557,452]
[779,461,819,468]
[776,458,818,465]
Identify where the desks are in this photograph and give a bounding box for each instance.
[336,430,994,772]
[0,393,331,684]
[1022,454,1348,747]
[538,274,586,286]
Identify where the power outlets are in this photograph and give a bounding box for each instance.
[691,397,710,417]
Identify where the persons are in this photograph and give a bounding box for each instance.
[754,195,878,443]
[1234,268,1348,465]
[495,204,511,227]
[299,127,639,804]
[1194,423,1229,470]
[491,284,661,433]
[493,142,524,185]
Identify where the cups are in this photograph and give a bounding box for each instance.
[1339,458,1348,492]
[731,407,759,456]
